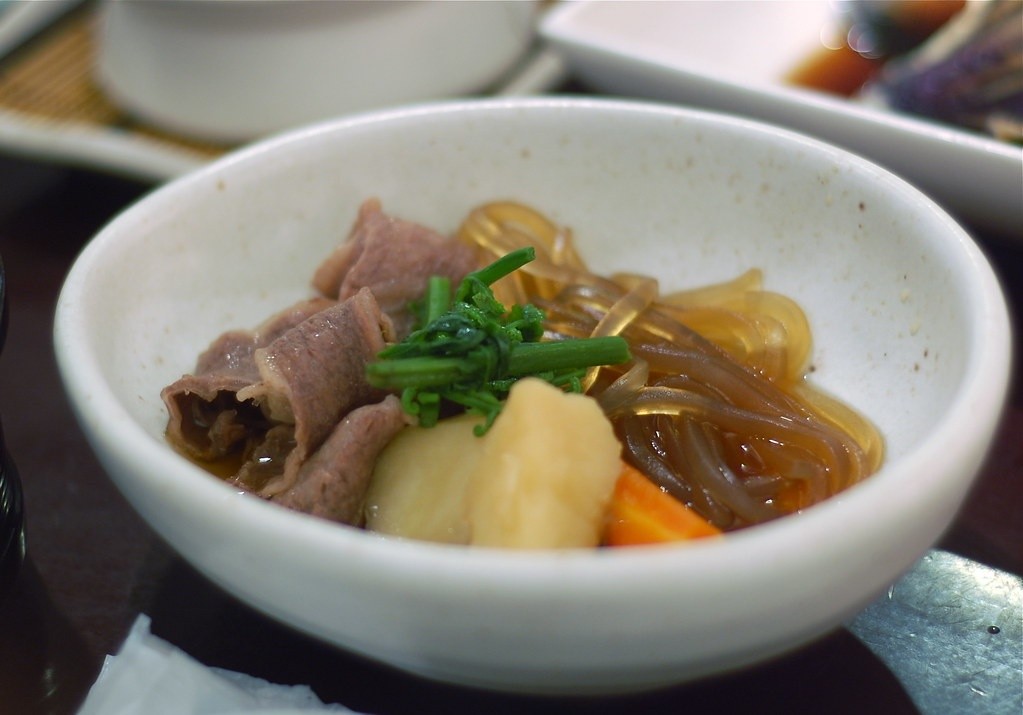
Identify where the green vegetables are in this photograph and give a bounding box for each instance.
[363,242,635,436]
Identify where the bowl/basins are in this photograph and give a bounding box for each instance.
[52,97,1014,696]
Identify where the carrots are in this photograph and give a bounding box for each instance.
[600,463,723,546]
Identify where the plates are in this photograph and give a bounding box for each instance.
[536,0,1023,228]
